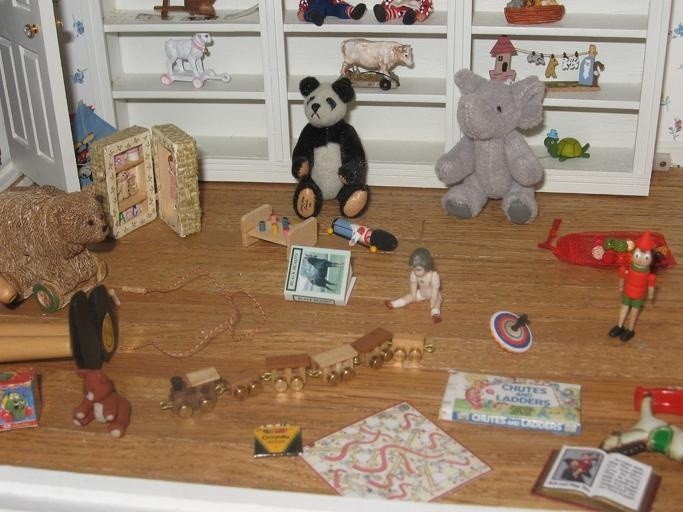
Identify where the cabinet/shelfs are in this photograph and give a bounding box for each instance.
[78,0,669,201]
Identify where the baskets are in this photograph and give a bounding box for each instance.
[504,5,565,25]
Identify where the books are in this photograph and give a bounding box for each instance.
[529,445,663,512]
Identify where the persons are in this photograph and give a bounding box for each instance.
[609,232,657,342]
[386,247,442,323]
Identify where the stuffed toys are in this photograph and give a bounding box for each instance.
[72,370,131,438]
[291,76,369,218]
[373,0,436,25]
[434,69,546,224]
[298,0,366,26]
